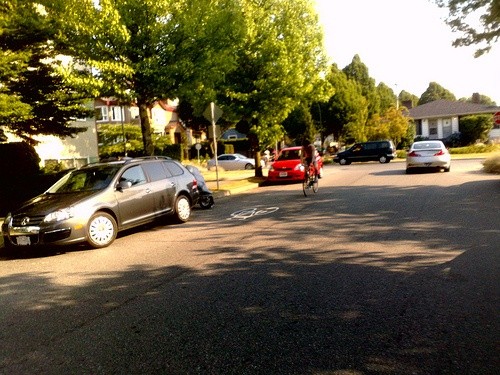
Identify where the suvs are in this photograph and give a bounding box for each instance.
[333,140,397,166]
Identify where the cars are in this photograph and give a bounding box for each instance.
[207,153,265,172]
[2,154,200,252]
[268,146,323,184]
[405,140,452,173]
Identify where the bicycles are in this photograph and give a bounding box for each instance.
[302,165,319,197]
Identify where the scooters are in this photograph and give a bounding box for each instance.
[196,184,214,210]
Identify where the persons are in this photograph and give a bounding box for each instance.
[300,140,322,179]
